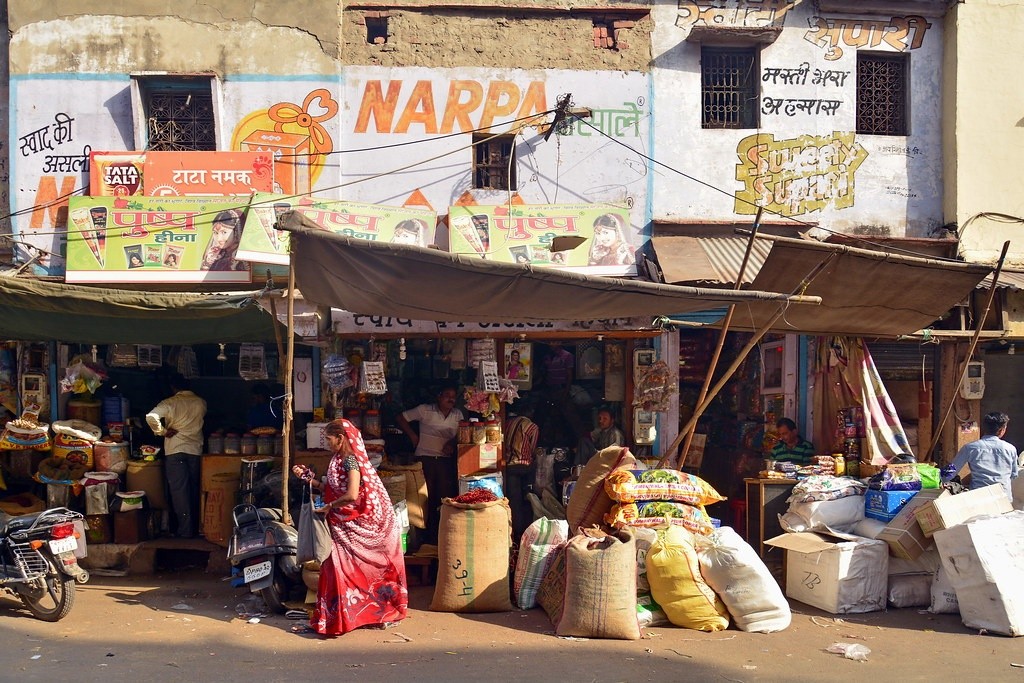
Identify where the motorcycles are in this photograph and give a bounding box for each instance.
[0,505,91,622]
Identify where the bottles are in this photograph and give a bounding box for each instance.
[208,433,283,457]
[458,414,502,444]
[346,410,381,440]
[845,424,861,478]
[832,453,845,477]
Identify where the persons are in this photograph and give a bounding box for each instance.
[199,209,252,271]
[391,218,423,249]
[291,419,408,637]
[938,410,1024,517]
[587,214,637,266]
[533,340,574,447]
[507,350,528,381]
[163,254,178,269]
[128,253,145,268]
[504,399,538,544]
[571,407,626,466]
[215,383,284,436]
[397,382,465,544]
[765,417,816,467]
[145,375,209,539]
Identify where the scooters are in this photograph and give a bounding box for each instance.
[227,466,312,615]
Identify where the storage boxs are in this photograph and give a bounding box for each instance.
[765,464,1024,622]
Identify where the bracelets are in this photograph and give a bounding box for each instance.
[329,501,333,508]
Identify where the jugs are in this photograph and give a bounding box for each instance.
[570,464,586,480]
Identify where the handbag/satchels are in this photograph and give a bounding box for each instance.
[295,481,332,562]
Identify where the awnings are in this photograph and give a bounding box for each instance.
[0,265,312,356]
[274,214,822,326]
[681,226,1024,342]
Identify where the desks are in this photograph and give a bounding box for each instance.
[745,477,800,566]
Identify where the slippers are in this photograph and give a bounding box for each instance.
[291,624,318,634]
